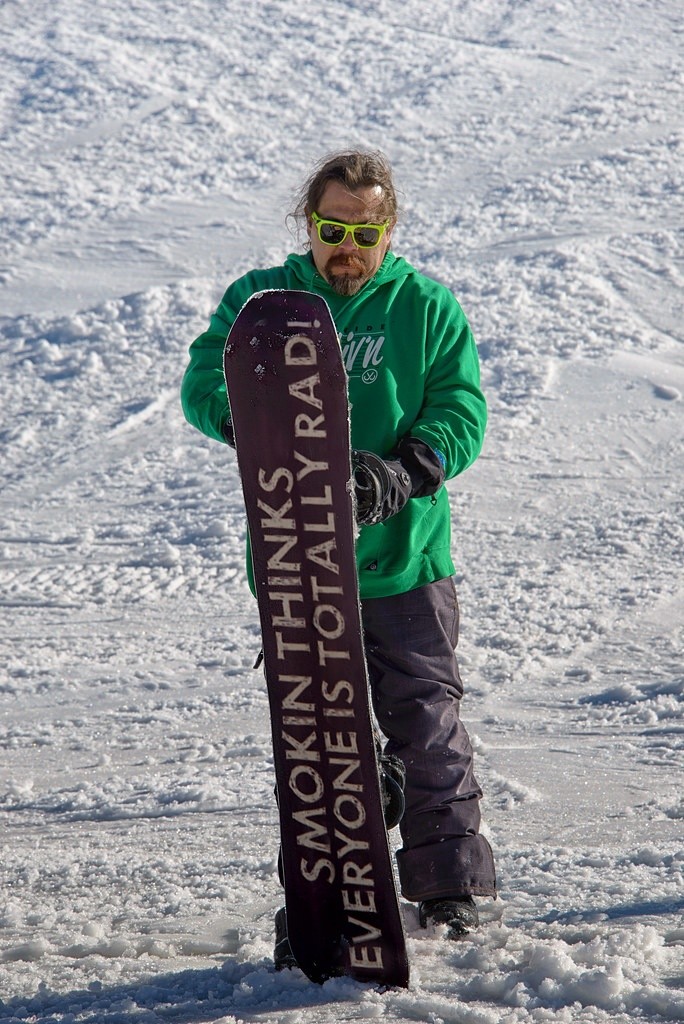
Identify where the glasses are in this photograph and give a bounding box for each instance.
[309,210,389,250]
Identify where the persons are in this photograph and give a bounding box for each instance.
[182,154,494,941]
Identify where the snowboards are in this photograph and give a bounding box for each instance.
[223,288,411,991]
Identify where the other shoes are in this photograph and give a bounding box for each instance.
[419,895,479,941]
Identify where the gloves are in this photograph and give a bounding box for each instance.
[352,440,445,527]
